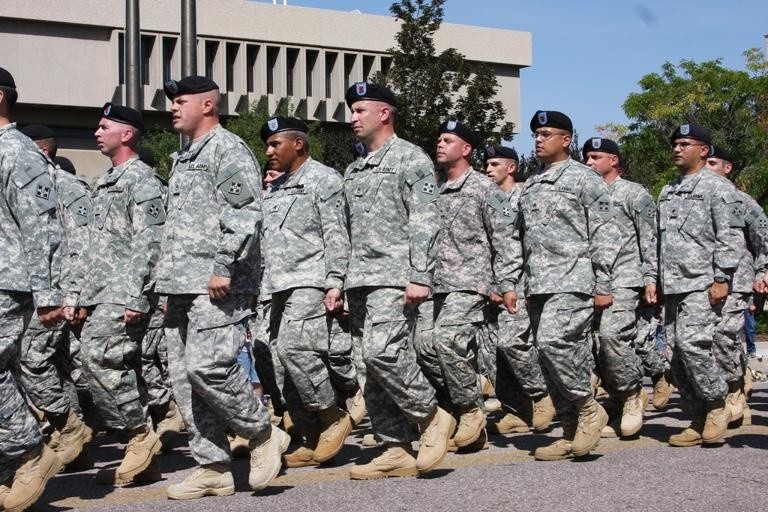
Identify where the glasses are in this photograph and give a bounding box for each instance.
[533,130,569,140]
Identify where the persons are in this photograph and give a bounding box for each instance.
[500,110,613,463]
[2,67,62,512]
[420,120,516,449]
[702,147,764,404]
[579,139,659,435]
[634,286,679,412]
[253,114,350,469]
[134,146,186,454]
[75,102,165,488]
[156,76,290,501]
[259,162,300,442]
[339,85,457,477]
[746,295,761,363]
[480,143,553,433]
[656,121,744,447]
[20,125,92,475]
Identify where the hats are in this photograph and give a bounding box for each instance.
[102,103,144,131]
[53,157,75,172]
[164,76,218,99]
[670,125,713,145]
[1,69,16,89]
[264,162,276,170]
[260,116,309,140]
[436,121,481,149]
[344,83,401,107]
[703,147,734,161]
[20,124,55,139]
[484,145,519,160]
[530,112,572,132]
[583,138,618,154]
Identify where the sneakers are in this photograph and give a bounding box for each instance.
[156,400,186,452]
[97,454,161,484]
[572,395,609,456]
[227,434,252,459]
[166,463,234,499]
[364,433,380,445]
[454,405,488,450]
[55,408,92,465]
[46,430,63,449]
[652,373,674,409]
[3,441,63,512]
[478,375,492,393]
[744,370,753,394]
[483,399,503,414]
[620,385,648,436]
[118,425,163,482]
[415,406,457,473]
[345,388,367,426]
[736,391,752,425]
[283,412,298,434]
[248,423,291,490]
[600,411,623,438]
[488,414,530,434]
[532,394,559,431]
[534,428,582,461]
[0,474,15,507]
[349,443,422,480]
[703,398,732,444]
[668,417,708,446]
[313,404,351,463]
[282,446,320,467]
